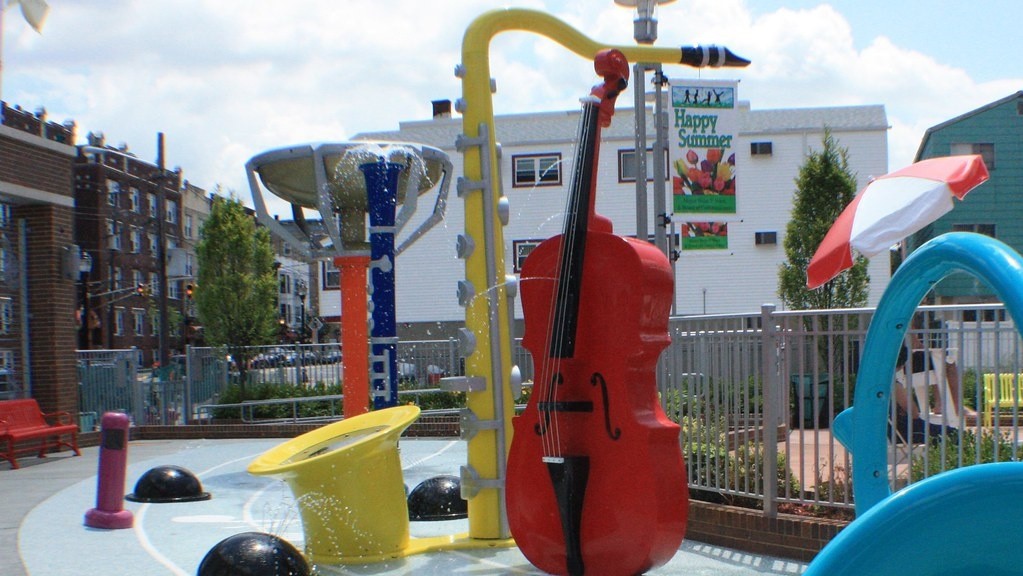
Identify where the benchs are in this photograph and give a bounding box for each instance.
[983,373,1023,429]
[0,398,83,469]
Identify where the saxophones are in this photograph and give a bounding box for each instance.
[248,6,751,569]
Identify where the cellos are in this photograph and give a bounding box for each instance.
[505,49,689,576]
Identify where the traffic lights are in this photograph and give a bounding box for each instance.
[186,283,193,300]
[137,283,144,297]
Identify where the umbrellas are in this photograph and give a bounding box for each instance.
[805,151,991,294]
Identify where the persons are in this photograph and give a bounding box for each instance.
[883,294,976,461]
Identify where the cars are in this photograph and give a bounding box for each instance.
[132,350,343,385]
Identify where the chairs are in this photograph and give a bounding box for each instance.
[888,414,927,482]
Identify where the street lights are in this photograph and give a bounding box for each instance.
[77,252,94,350]
[298,282,309,382]
[615,0,674,257]
